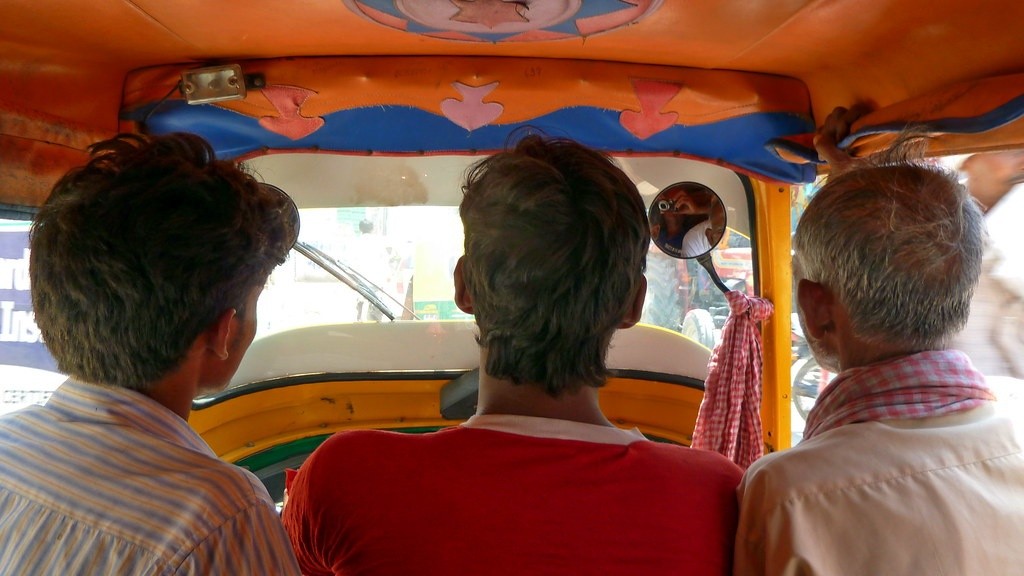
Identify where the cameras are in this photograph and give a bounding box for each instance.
[658,199,679,212]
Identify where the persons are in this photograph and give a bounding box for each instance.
[731,119,1024,576]
[0,130,299,576]
[282,127,743,576]
[648,184,724,258]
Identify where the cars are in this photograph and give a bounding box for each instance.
[650,220,757,309]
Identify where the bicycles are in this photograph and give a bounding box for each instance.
[796,355,843,422]
